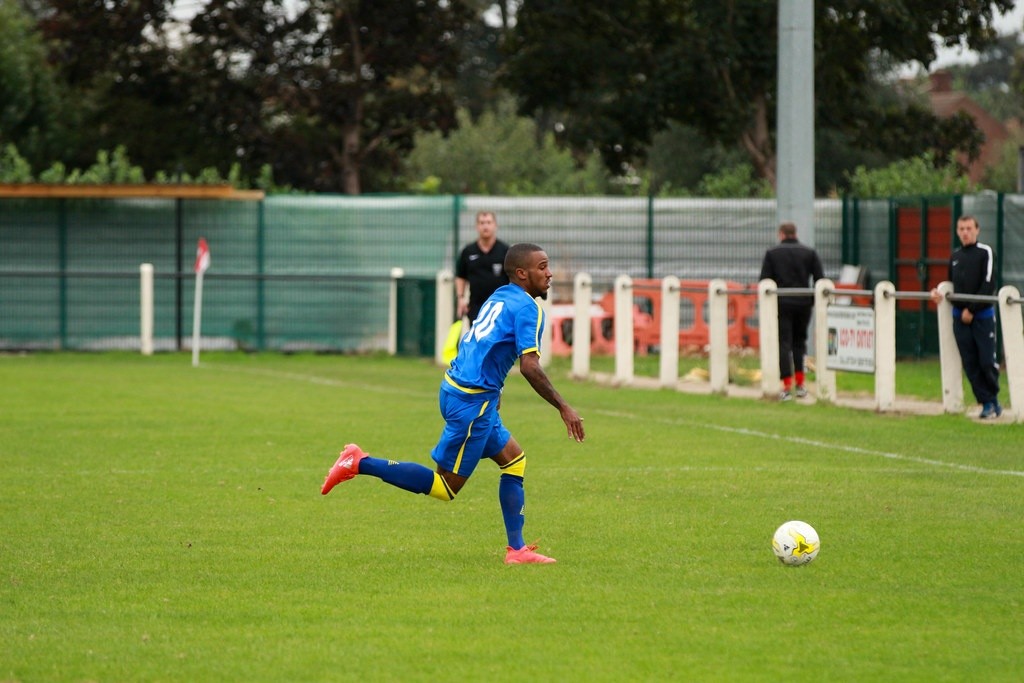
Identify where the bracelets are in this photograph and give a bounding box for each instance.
[456,295,464,299]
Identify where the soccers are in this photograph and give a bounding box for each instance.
[772,521,820,567]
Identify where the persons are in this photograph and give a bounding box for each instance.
[930,215,1002,417]
[759,222,825,403]
[321,242,586,564]
[454,209,512,331]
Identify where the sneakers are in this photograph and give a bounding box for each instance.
[322,443,369,494]
[503,544,557,565]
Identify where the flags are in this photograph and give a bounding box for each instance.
[194,238,211,274]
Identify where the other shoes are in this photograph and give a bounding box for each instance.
[795,389,815,406]
[777,390,791,402]
[980,403,1003,420]
